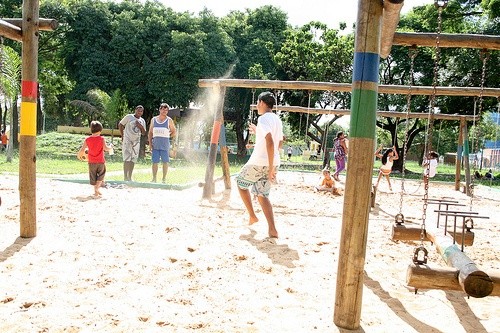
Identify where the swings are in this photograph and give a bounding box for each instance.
[425,120,443,179]
[379,116,399,174]
[301,91,335,182]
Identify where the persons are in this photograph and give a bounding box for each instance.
[118,105,147,182]
[0,130,9,152]
[287,145,293,161]
[331,131,348,181]
[422,150,438,193]
[148,103,177,184]
[77,121,114,197]
[320,169,335,188]
[374,145,399,191]
[474,169,499,180]
[236,91,283,237]
